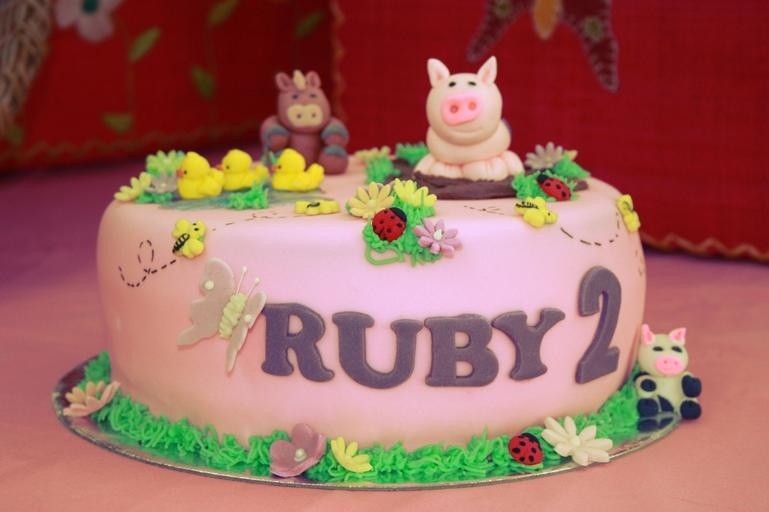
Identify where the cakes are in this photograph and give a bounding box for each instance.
[61,54,702,482]
